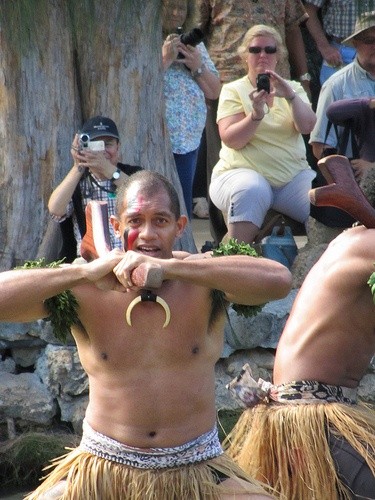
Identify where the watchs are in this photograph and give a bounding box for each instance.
[190,63,205,77]
[109,169,121,182]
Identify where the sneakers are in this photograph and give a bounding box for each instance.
[191,197,210,219]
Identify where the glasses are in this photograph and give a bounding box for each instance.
[245,45,278,54]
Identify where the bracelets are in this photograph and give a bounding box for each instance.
[251,111,264,121]
[284,90,297,100]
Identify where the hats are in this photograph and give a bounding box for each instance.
[340,11,375,45]
[79,117,119,141]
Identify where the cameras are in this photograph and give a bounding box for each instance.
[257,74,271,95]
[175,27,203,59]
[78,134,105,152]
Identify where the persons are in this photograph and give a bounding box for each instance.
[163,0,220,227]
[209,25,317,247]
[311,9,375,226]
[48,116,142,264]
[191,130,210,219]
[1,172,292,500]
[305,0,375,85]
[209,1,313,102]
[226,221,374,499]
